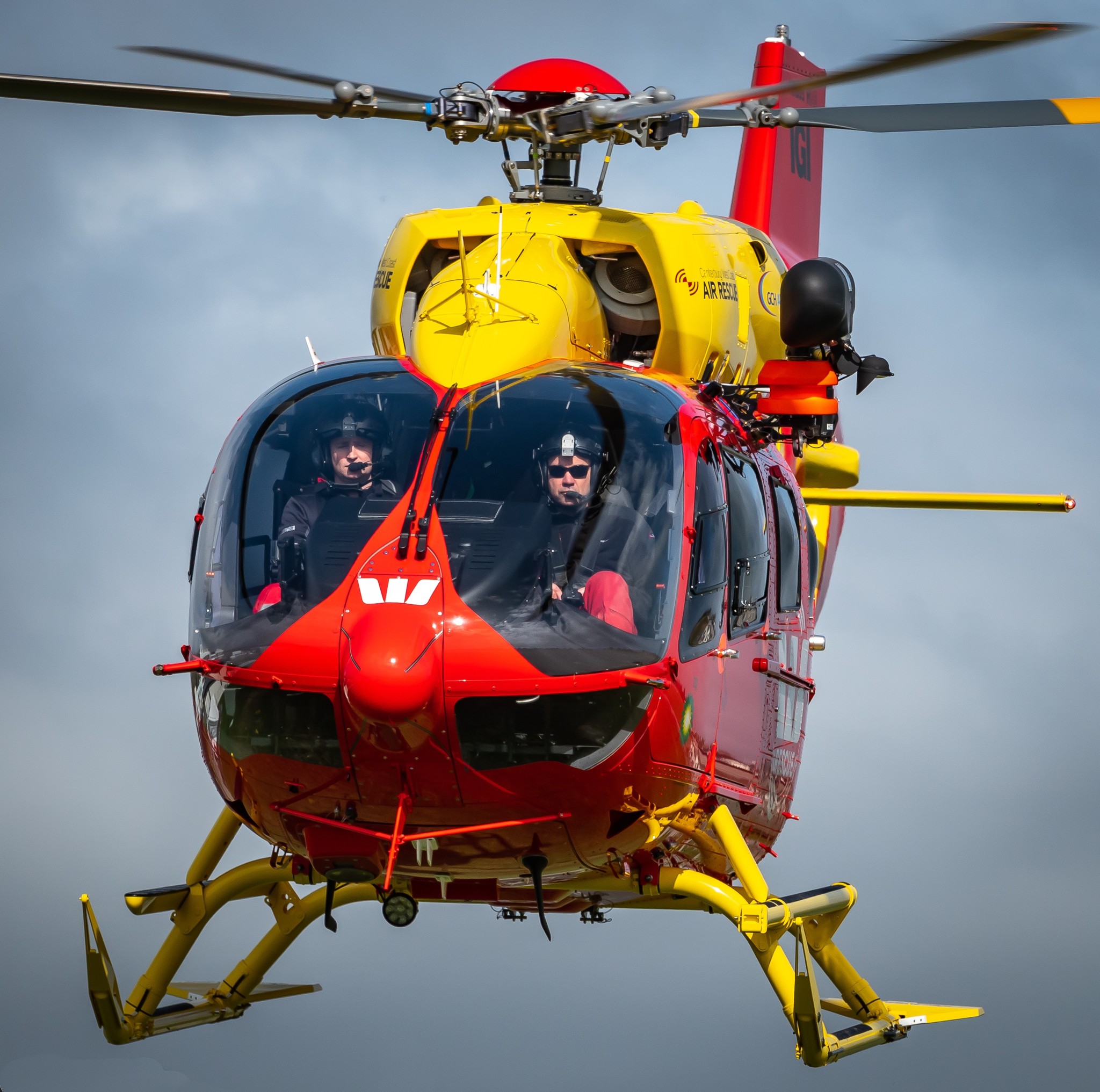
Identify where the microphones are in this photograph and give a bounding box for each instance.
[566,491,597,502]
[349,460,382,472]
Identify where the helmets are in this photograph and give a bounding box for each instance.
[532,427,608,489]
[312,401,392,468]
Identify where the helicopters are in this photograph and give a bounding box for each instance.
[0,10,1100,1071]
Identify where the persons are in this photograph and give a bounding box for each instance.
[275,399,403,571]
[499,420,655,635]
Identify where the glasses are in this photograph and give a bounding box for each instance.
[546,462,592,479]
